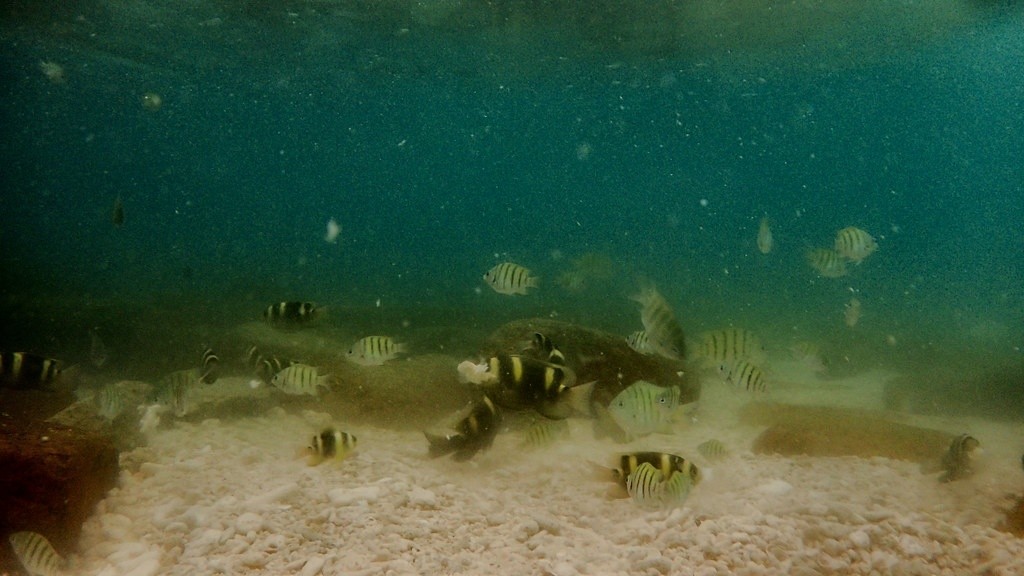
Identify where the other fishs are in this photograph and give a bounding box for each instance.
[0,211,981,576]
